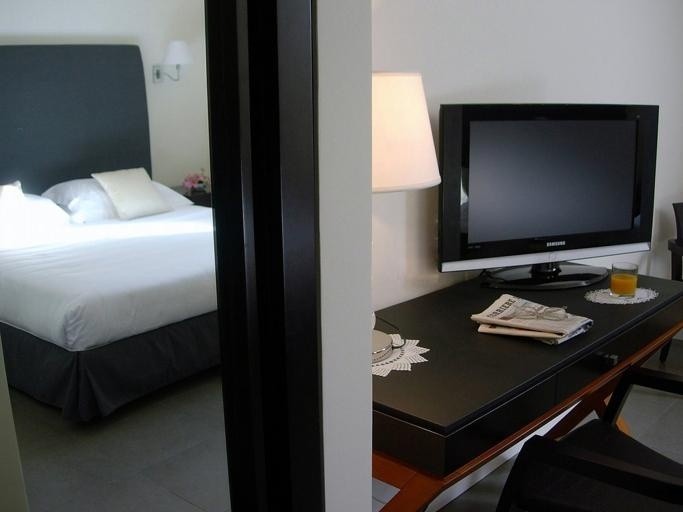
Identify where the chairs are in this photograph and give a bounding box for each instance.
[660,202,683,363]
[497,366,683,512]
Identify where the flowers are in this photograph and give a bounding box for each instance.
[183,175,208,189]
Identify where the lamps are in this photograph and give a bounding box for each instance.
[372,72,441,362]
[152,41,192,82]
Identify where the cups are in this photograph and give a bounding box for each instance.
[609,262,638,298]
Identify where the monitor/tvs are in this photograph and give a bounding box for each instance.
[438,103,659,291]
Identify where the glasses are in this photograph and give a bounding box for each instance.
[511,303,568,321]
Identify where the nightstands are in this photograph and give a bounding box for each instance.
[172,185,211,207]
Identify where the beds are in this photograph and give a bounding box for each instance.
[0,44,218,430]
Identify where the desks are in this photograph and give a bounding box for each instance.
[372,261,683,512]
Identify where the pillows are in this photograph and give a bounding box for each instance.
[0,180,69,251]
[42,178,193,222]
[91,167,171,220]
